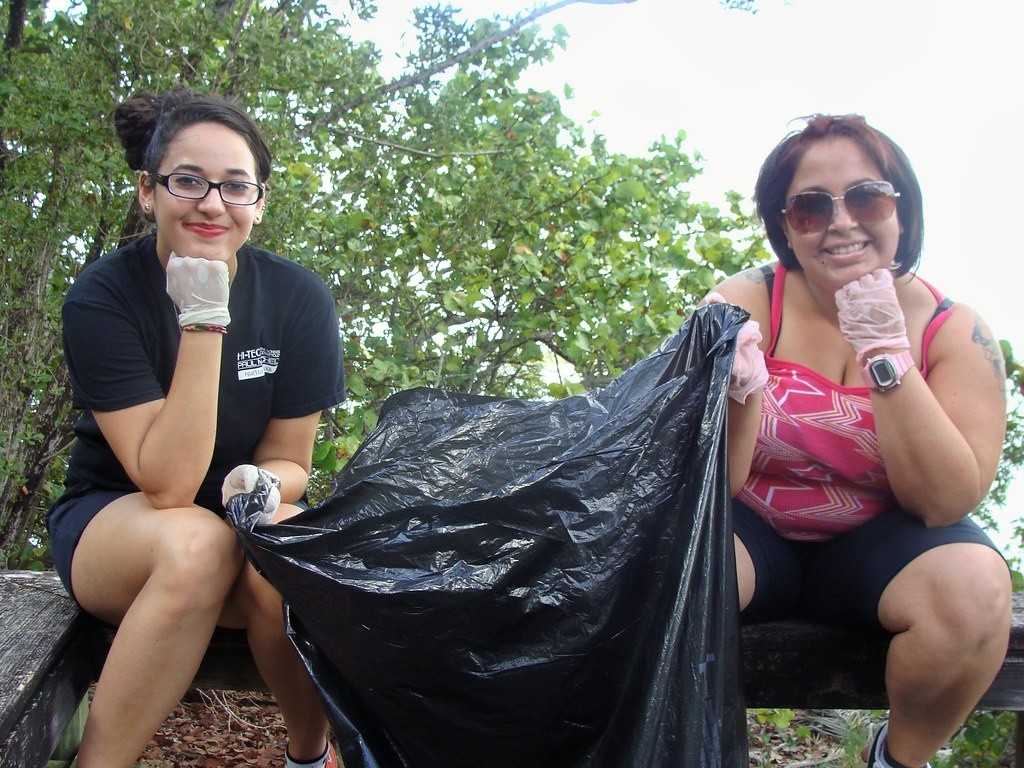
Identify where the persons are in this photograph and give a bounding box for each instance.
[47,83,346,768]
[687,113,1011,768]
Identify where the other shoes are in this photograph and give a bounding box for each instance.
[323,740,338,768]
[867,722,932,768]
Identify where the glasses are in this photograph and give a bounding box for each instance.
[149,173,263,206]
[781,181,900,235]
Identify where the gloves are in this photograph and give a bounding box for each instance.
[220,464,281,526]
[166,251,231,326]
[833,271,911,365]
[688,293,769,404]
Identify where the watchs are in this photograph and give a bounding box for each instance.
[860,350,914,395]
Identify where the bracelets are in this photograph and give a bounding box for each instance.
[180,323,227,334]
